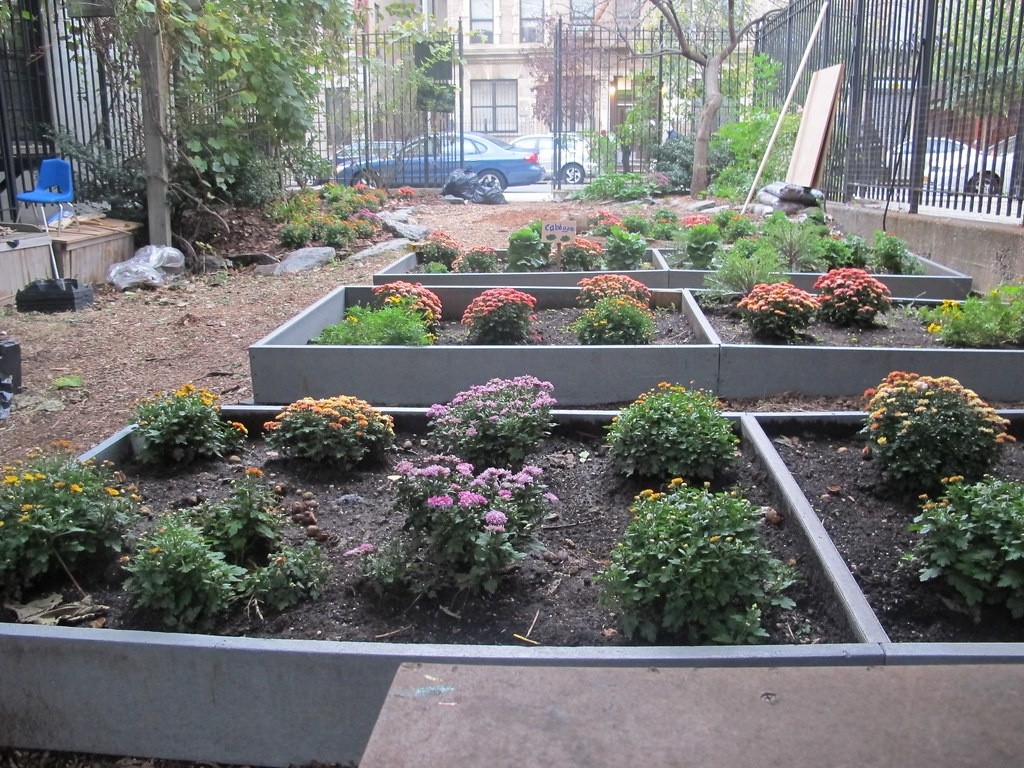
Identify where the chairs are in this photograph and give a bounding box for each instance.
[14,158,80,237]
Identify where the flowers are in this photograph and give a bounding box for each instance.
[421,229,502,273]
[561,238,604,271]
[459,289,543,345]
[646,171,669,187]
[926,288,1023,348]
[2,439,129,579]
[899,472,1024,619]
[356,454,550,614]
[260,395,397,465]
[344,280,442,346]
[281,181,417,249]
[601,381,733,487]
[129,381,247,458]
[812,267,893,328]
[736,282,823,338]
[575,274,652,346]
[859,370,1016,498]
[678,214,712,230]
[125,464,329,632]
[600,478,798,648]
[427,374,557,467]
[586,210,628,237]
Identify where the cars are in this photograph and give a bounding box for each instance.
[503,131,605,183]
[332,131,544,195]
[882,135,980,181]
[927,134,1018,197]
[317,137,397,168]
